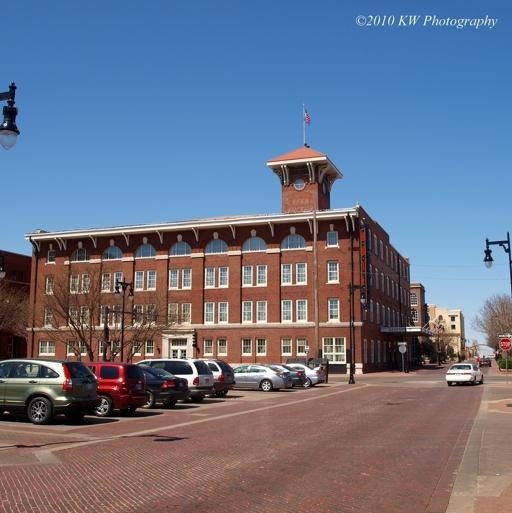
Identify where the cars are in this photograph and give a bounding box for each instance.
[444,363,484,386]
[138,364,188,410]
[231,362,326,392]
[478,357,491,367]
[198,358,236,398]
[0,357,101,426]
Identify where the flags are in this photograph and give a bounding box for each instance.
[304,106,311,125]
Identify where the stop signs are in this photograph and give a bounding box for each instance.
[499,337,512,350]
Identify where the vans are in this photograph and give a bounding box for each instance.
[85,361,151,417]
[136,358,215,403]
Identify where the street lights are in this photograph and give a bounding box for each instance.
[481,233,512,290]
[115,277,135,362]
[348,280,369,384]
